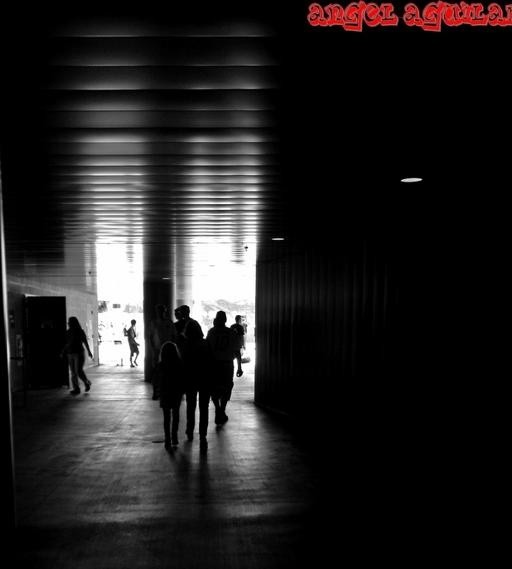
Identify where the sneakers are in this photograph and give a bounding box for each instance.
[85,380,91,391]
[70,387,80,394]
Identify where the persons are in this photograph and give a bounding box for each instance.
[115,305,249,448]
[61,317,92,394]
